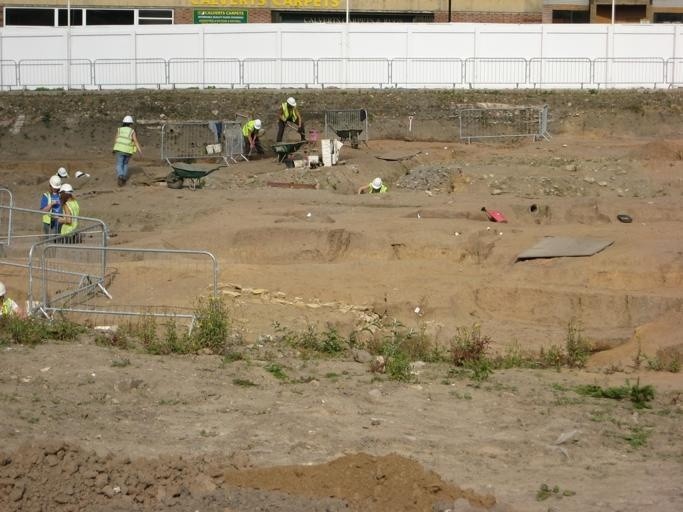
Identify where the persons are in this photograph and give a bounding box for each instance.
[240,119,265,161]
[275,97,305,144]
[38,175,64,246]
[0,283,22,317]
[111,116,142,186]
[54,166,70,178]
[74,171,90,178]
[356,177,388,196]
[56,184,80,246]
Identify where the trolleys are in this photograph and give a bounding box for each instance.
[162,156,226,191]
[255,141,308,163]
[327,124,362,149]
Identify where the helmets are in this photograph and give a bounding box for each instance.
[122,116,134,124]
[0,282,6,296]
[48,167,75,192]
[75,171,84,178]
[370,177,383,189]
[254,119,262,129]
[287,97,297,107]
